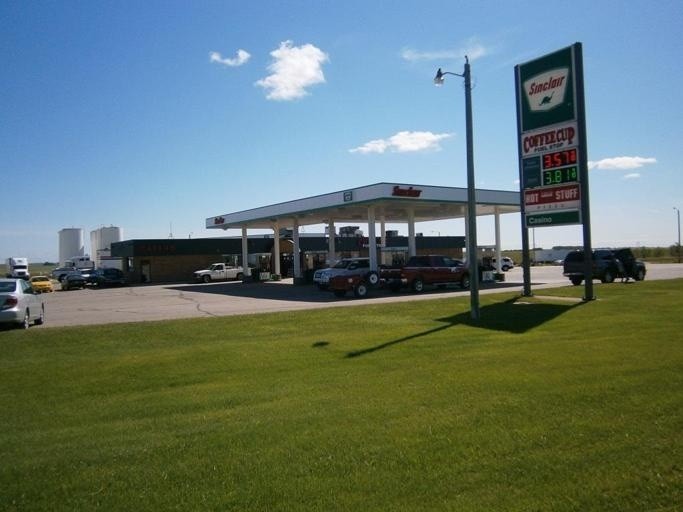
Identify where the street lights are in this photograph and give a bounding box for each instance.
[431,54,479,319]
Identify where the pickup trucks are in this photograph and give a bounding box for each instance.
[193,262,255,283]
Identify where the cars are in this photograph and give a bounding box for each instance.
[313,254,471,298]
[52,256,128,291]
[0,275,53,329]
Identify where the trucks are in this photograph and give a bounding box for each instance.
[492,256,514,271]
[7,257,30,280]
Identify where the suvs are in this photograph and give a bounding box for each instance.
[563,247,646,285]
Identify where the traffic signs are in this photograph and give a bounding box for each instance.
[673,206,681,247]
